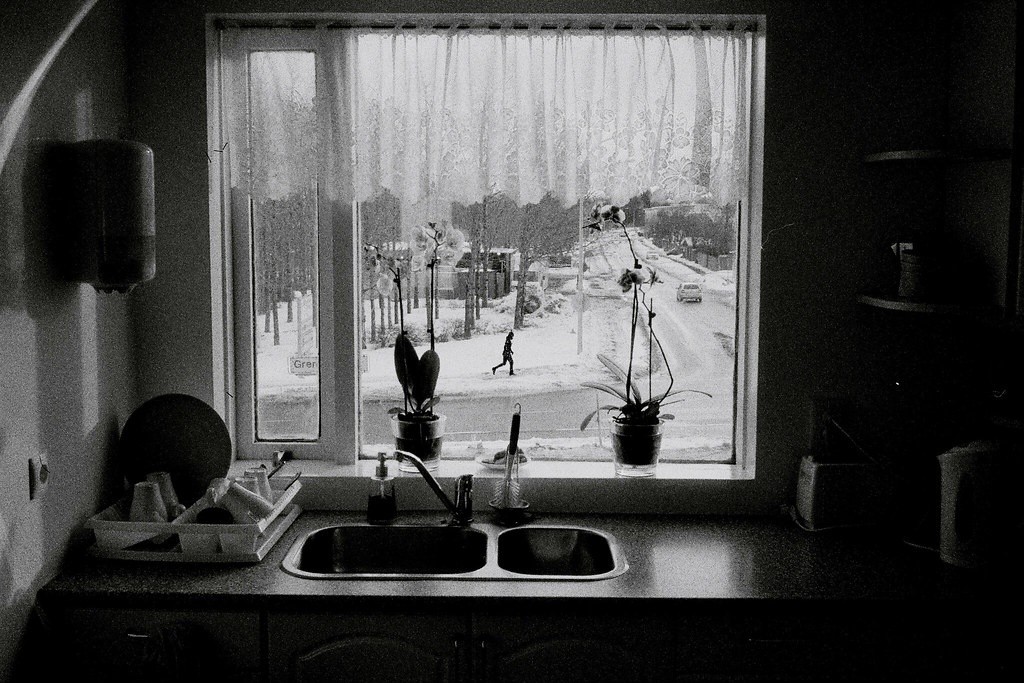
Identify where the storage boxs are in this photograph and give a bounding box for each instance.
[84,475,303,553]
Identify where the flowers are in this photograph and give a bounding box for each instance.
[578,207,713,433]
[362,220,468,415]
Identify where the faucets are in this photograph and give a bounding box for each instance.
[393,450,475,525]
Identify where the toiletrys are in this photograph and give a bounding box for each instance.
[366,451,395,525]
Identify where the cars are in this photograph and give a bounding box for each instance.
[675,282,703,302]
[644,249,661,261]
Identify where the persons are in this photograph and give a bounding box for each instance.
[492,331,515,376]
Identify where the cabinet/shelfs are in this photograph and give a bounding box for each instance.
[55,605,1024,683]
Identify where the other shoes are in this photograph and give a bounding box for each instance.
[492,367,496,375]
[509,373,515,375]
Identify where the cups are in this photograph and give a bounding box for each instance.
[130,481,168,522]
[207,469,273,525]
[146,472,178,510]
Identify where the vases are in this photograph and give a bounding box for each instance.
[610,419,662,477]
[391,413,447,472]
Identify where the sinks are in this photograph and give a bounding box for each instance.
[493,524,629,582]
[280,523,493,581]
